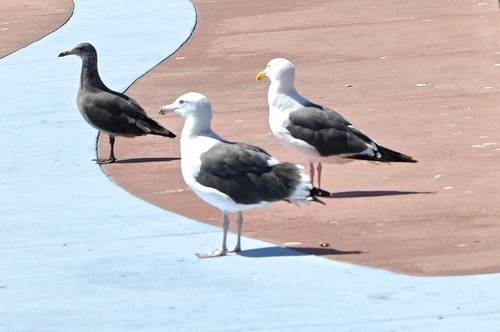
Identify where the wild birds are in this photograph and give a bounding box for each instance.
[256,57,419,197]
[56,43,177,165]
[158,92,333,259]
